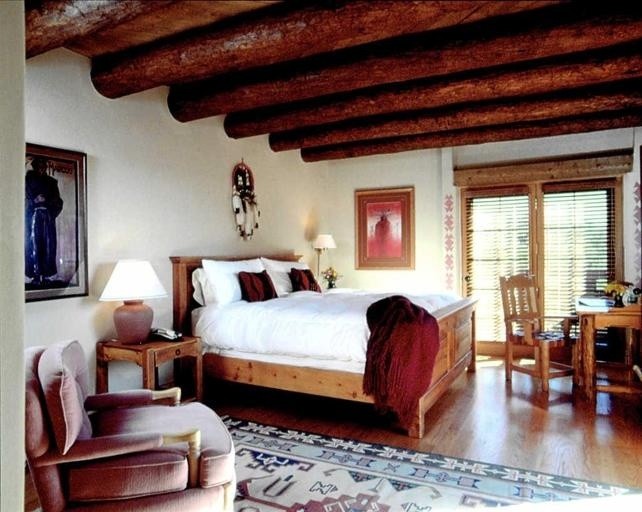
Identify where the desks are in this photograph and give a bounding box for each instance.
[569,301,641,406]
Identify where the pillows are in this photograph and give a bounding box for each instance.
[288,266,321,294]
[200,256,267,306]
[236,269,279,304]
[259,252,310,296]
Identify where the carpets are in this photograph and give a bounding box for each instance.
[218,406,642,512]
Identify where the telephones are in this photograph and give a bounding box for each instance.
[150,327,182,342]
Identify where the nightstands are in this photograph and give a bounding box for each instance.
[96,336,204,404]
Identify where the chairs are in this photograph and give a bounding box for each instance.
[497,271,582,405]
[25,339,238,512]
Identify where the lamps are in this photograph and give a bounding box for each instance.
[99,257,172,345]
[312,231,337,256]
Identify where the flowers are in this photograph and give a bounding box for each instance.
[599,279,630,297]
[320,265,346,282]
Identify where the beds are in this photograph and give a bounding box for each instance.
[169,248,479,442]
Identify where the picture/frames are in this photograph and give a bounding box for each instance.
[352,184,417,272]
[22,141,90,304]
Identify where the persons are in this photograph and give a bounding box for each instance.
[24,160,63,287]
[374,215,393,242]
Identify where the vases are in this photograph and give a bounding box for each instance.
[611,294,626,311]
[325,279,337,289]
